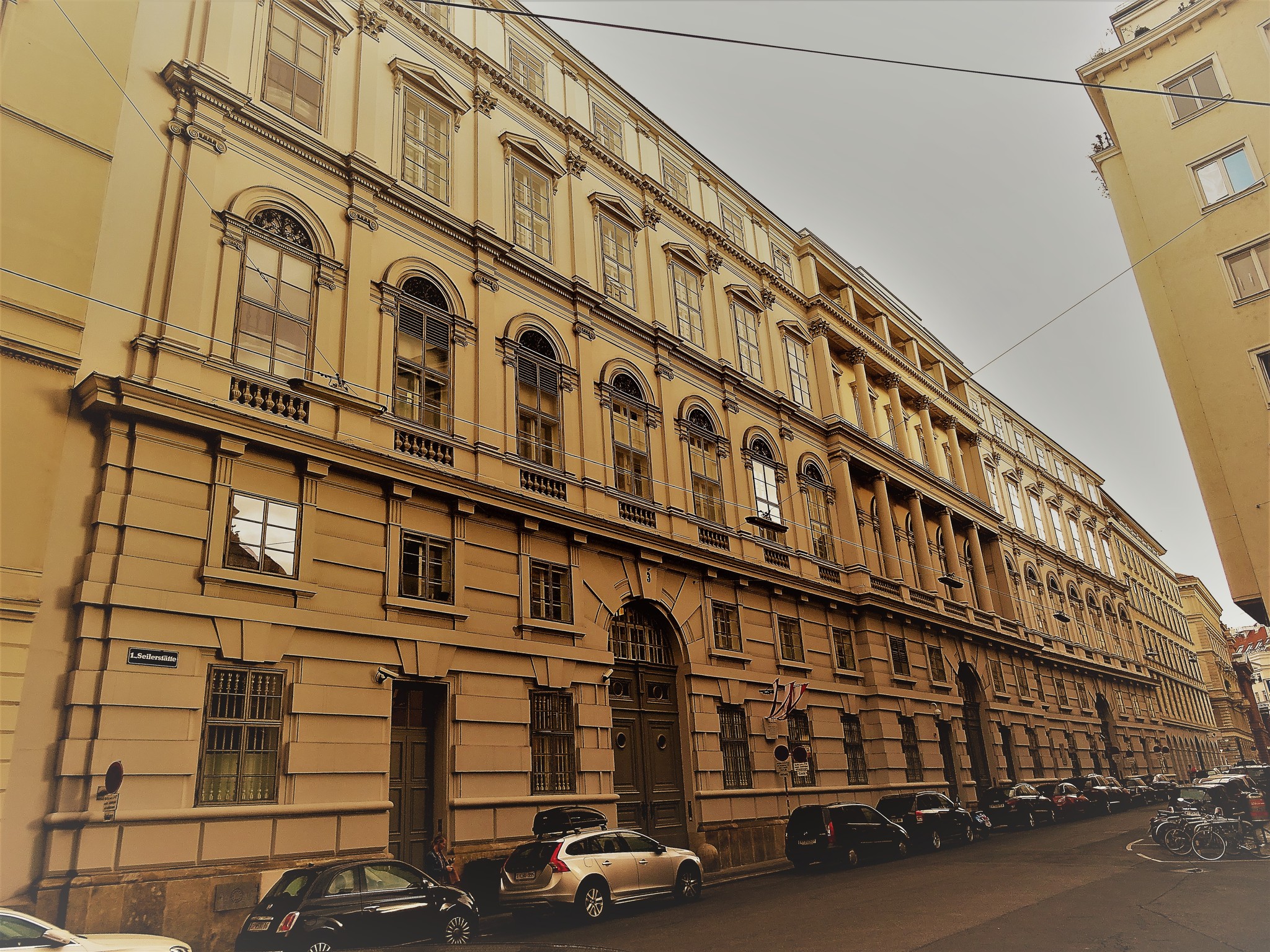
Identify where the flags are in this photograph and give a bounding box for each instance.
[764,677,808,721]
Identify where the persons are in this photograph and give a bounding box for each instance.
[955,793,960,805]
[1187,764,1196,783]
[427,836,453,884]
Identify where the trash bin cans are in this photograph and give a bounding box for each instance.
[466,856,505,916]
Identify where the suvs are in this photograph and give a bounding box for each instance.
[783,801,913,871]
[977,782,1056,831]
[1063,772,1182,814]
[497,805,704,927]
[874,790,974,852]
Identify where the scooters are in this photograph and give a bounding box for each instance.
[1147,789,1210,846]
[952,793,993,841]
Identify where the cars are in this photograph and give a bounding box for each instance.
[1166,759,1270,820]
[0,904,194,952]
[1036,783,1090,823]
[235,857,480,952]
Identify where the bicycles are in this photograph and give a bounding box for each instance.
[1155,811,1270,860]
